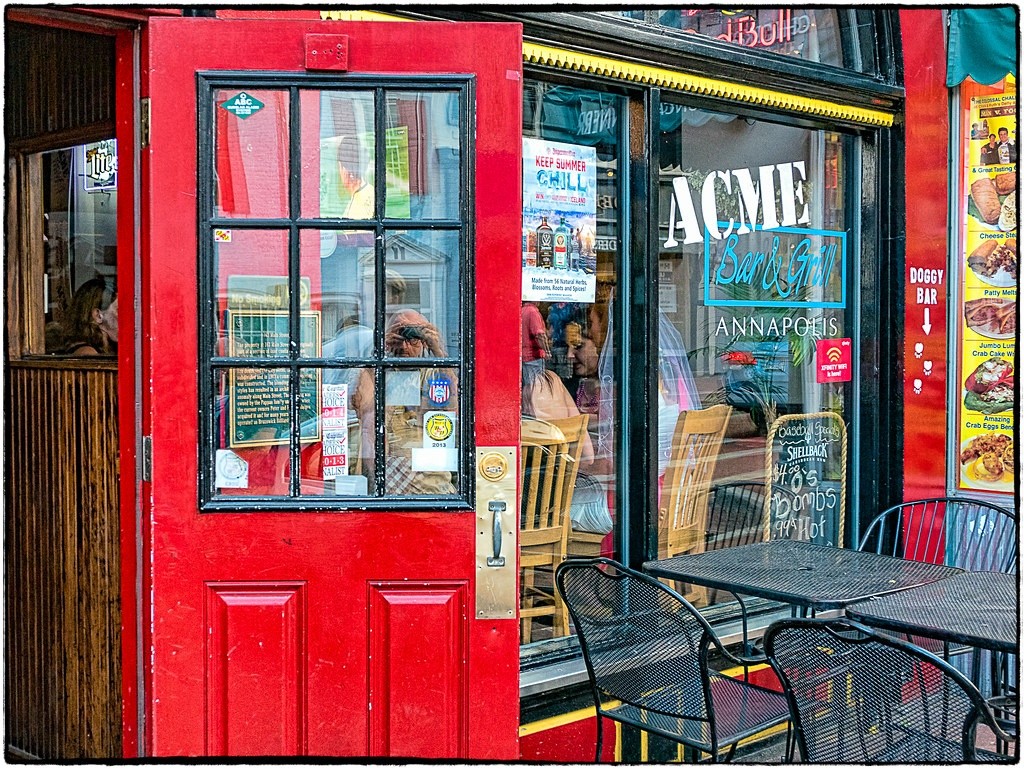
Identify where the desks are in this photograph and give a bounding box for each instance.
[642,538,964,765]
[844,570,1018,763]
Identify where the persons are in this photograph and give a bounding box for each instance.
[337,136,374,233]
[44,274,119,355]
[521,283,707,535]
[301,307,458,496]
[971,123,1017,166]
[521,300,552,419]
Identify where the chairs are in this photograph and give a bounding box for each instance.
[554,555,827,764]
[761,615,1016,764]
[809,495,1017,761]
[567,403,733,613]
[520,413,588,645]
[680,479,801,607]
